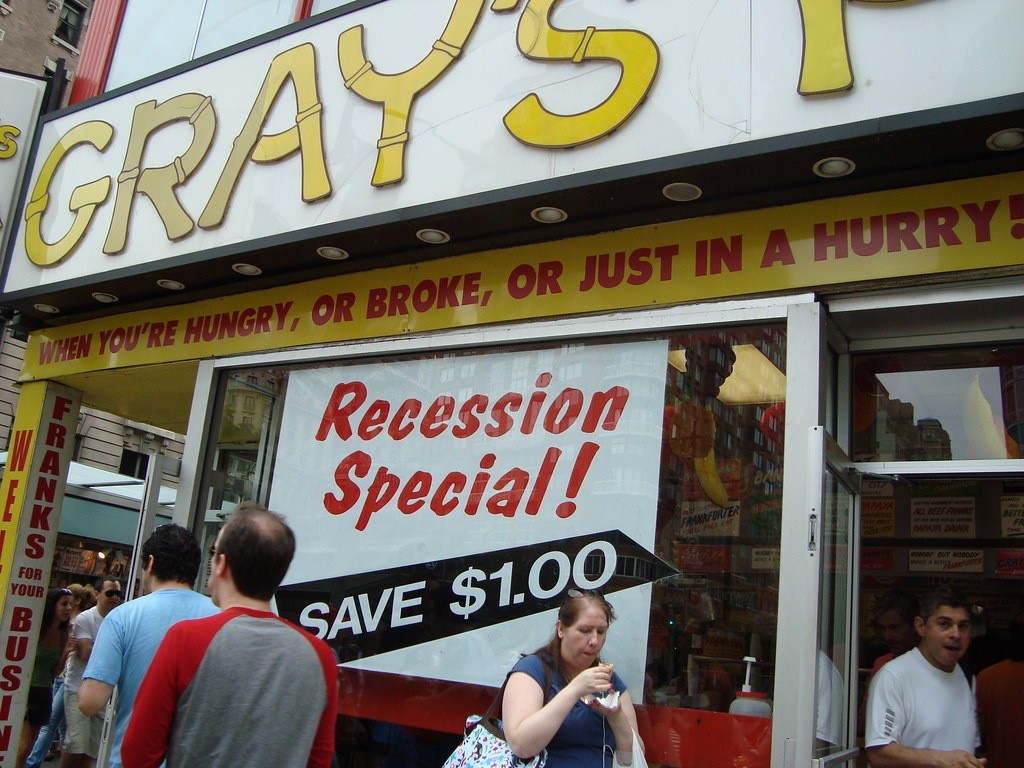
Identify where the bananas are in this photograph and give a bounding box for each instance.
[694,445,730,509]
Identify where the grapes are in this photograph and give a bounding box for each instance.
[684,341,736,397]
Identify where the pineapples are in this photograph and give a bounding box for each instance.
[669,398,716,458]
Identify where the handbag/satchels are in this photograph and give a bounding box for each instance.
[442,652,552,768]
[613,728,648,768]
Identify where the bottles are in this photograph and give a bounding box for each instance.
[728,691,771,717]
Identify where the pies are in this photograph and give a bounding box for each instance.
[598,663,613,681]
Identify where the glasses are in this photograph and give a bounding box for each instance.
[568,587,603,598]
[208,545,222,556]
[153,522,182,533]
[56,588,72,595]
[99,590,123,598]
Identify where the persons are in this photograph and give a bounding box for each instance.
[858,589,1024,768]
[663,614,702,683]
[501,587,645,768]
[14,523,220,768]
[814,648,843,755]
[119,500,341,768]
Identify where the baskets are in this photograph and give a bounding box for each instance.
[703,634,744,658]
